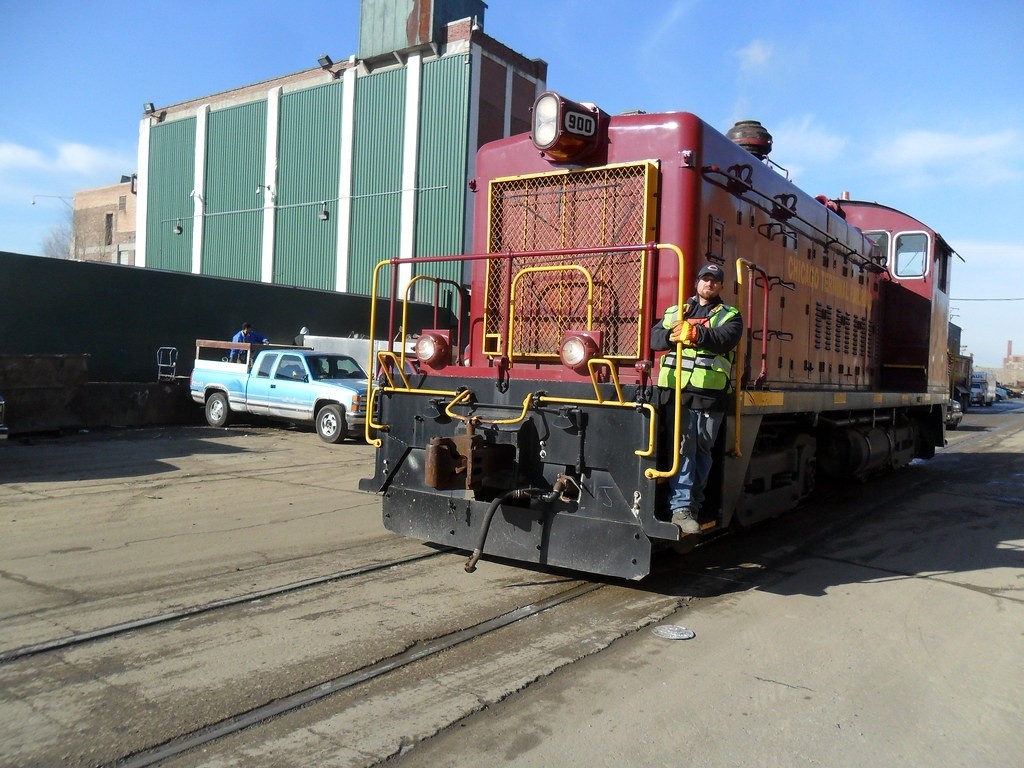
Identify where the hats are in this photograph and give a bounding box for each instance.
[299,326,310,335]
[697,264,724,284]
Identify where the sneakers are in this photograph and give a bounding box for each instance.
[689,509,698,522]
[673,510,701,534]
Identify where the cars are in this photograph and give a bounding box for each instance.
[996,381,1024,402]
[947,399,962,429]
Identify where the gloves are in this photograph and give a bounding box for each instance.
[680,339,698,349]
[673,321,700,342]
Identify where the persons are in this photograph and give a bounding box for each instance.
[293,327,310,346]
[649,265,743,534]
[228,322,269,364]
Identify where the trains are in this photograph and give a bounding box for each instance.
[359,93,964,582]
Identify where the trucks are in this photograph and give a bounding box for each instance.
[971,371,997,406]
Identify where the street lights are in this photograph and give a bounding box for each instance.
[31,194,73,210]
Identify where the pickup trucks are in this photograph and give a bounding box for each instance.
[191,339,379,444]
